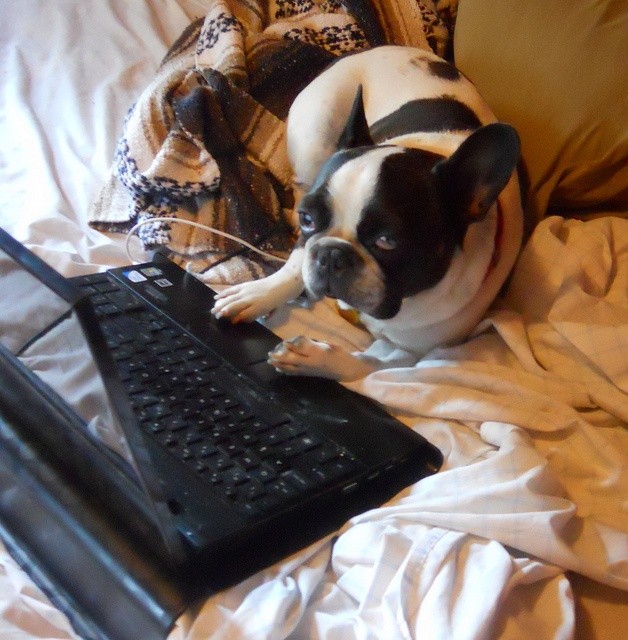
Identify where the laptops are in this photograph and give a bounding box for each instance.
[0,227,442,640]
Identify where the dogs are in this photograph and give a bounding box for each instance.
[209,45,532,377]
